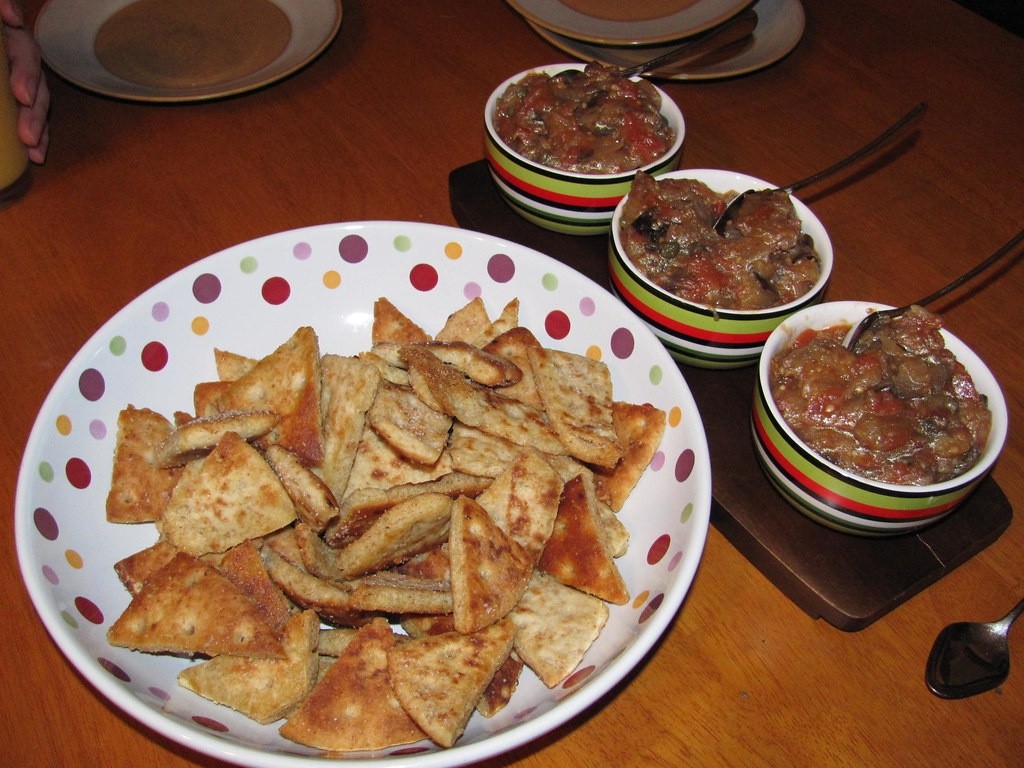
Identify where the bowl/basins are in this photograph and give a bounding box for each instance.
[603,168,835,369]
[751,299,1010,538]
[483,62,686,236]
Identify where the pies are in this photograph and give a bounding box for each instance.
[104,296,666,753]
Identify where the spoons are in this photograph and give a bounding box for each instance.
[924,592,1024,700]
[847,232,1022,353]
[713,102,925,235]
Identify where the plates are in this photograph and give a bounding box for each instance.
[11,217,713,768]
[527,0,806,82]
[28,0,346,103]
[504,0,759,46]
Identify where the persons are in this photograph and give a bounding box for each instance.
[0,0,50,164]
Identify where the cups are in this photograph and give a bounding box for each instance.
[0,26,32,190]
